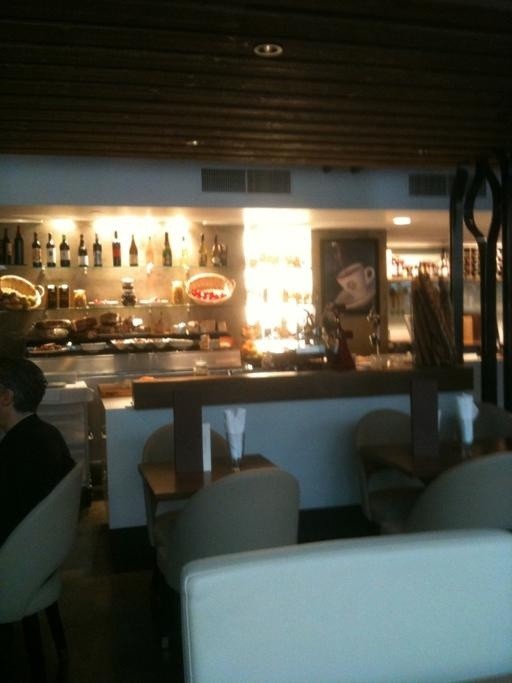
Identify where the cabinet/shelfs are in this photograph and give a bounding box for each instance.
[386,243,503,344]
[0,215,247,397]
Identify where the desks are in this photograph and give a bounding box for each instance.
[138,453,281,553]
[359,440,485,526]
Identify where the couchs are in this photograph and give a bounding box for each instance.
[180,527,511,682]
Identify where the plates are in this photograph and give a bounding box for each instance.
[333,279,376,311]
[27,346,73,354]
[87,300,118,307]
[139,298,168,306]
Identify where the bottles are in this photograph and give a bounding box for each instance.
[0,223,227,266]
[73,287,87,307]
[120,276,137,307]
[171,278,184,305]
[193,359,208,375]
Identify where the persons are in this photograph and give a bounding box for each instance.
[0,358,75,547]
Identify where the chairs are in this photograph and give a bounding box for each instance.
[404,450,511,530]
[0,458,85,683]
[156,466,300,596]
[356,409,412,523]
[143,422,228,546]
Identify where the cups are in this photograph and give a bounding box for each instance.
[227,430,246,472]
[336,261,376,300]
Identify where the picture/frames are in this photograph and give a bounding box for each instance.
[320,238,379,315]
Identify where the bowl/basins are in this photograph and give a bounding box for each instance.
[79,342,107,354]
[105,337,169,352]
[169,338,193,350]
